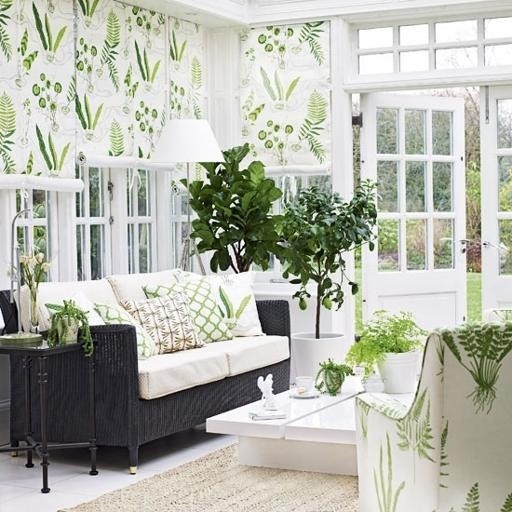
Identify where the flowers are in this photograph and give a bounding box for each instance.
[14,254,52,323]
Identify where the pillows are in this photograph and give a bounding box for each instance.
[173,273,265,335]
[95,303,158,360]
[120,298,205,353]
[141,281,234,341]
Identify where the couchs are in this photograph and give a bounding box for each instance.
[0,273,292,474]
[353,324,512,511]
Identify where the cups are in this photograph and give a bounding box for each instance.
[295,376,315,391]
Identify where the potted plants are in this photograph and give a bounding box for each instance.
[45,301,94,357]
[268,180,379,379]
[349,311,428,395]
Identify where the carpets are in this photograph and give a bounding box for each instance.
[60,443,357,512]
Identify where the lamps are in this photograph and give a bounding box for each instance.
[151,119,225,271]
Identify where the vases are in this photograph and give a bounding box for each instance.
[27,289,39,334]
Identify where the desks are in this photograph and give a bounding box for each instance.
[4,336,98,493]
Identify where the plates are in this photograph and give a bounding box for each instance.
[289,390,318,399]
